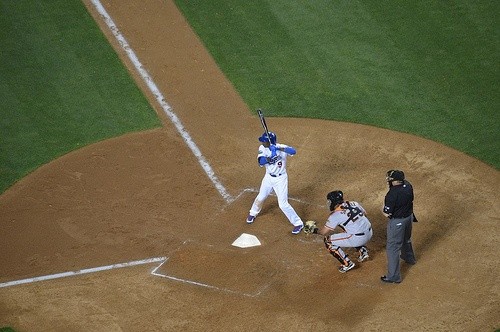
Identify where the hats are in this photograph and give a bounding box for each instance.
[387,168,404,179]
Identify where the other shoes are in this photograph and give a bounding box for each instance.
[338,260,355,274]
[246,215,255,223]
[292,225,304,234]
[381,275,399,284]
[357,247,369,262]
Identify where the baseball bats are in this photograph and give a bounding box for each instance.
[257,109,272,146]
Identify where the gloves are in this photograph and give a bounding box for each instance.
[268,145,278,151]
[270,153,277,161]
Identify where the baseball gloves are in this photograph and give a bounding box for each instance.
[301,220,319,237]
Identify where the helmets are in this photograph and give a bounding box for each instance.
[325,190,344,210]
[258,132,276,144]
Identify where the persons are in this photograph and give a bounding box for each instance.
[246,131,304,234]
[380,170,417,283]
[304,191,373,273]
